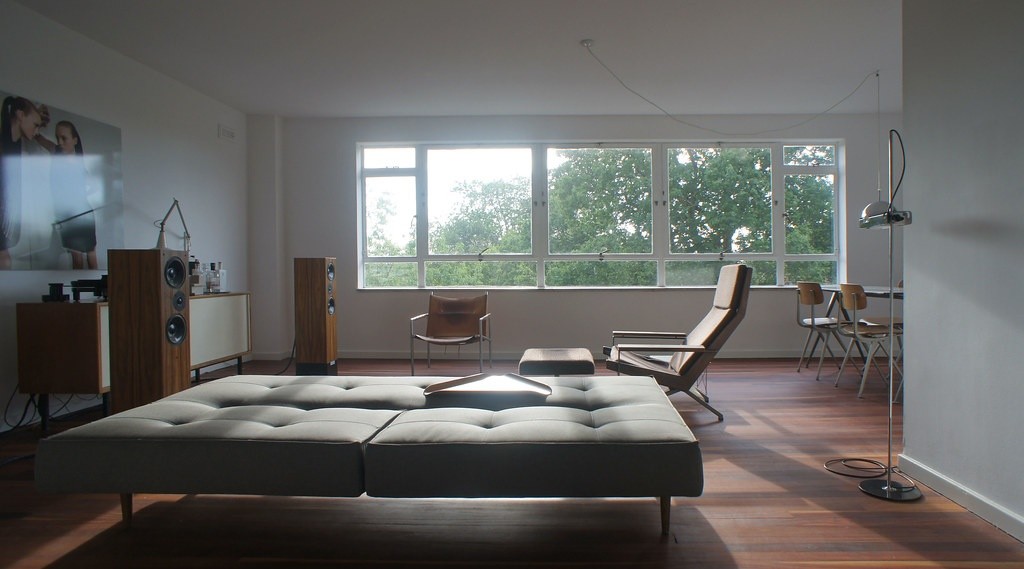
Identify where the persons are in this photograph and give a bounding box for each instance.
[35,104,100,270]
[0,97,41,270]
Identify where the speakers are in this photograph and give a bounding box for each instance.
[294,258,338,377]
[108,247,191,414]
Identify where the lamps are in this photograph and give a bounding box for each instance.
[860,71,896,230]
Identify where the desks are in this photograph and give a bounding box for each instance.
[805,284,903,370]
[16,292,252,431]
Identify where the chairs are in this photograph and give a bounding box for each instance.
[836,284,903,404]
[600,264,753,427]
[795,282,867,385]
[407,292,493,374]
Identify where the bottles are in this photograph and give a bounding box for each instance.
[209,263,220,293]
[189,255,199,275]
[218,262,226,293]
[202,263,210,292]
[192,258,203,295]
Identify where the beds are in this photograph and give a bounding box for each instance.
[33,376,702,539]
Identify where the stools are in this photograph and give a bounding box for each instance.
[519,347,596,377]
